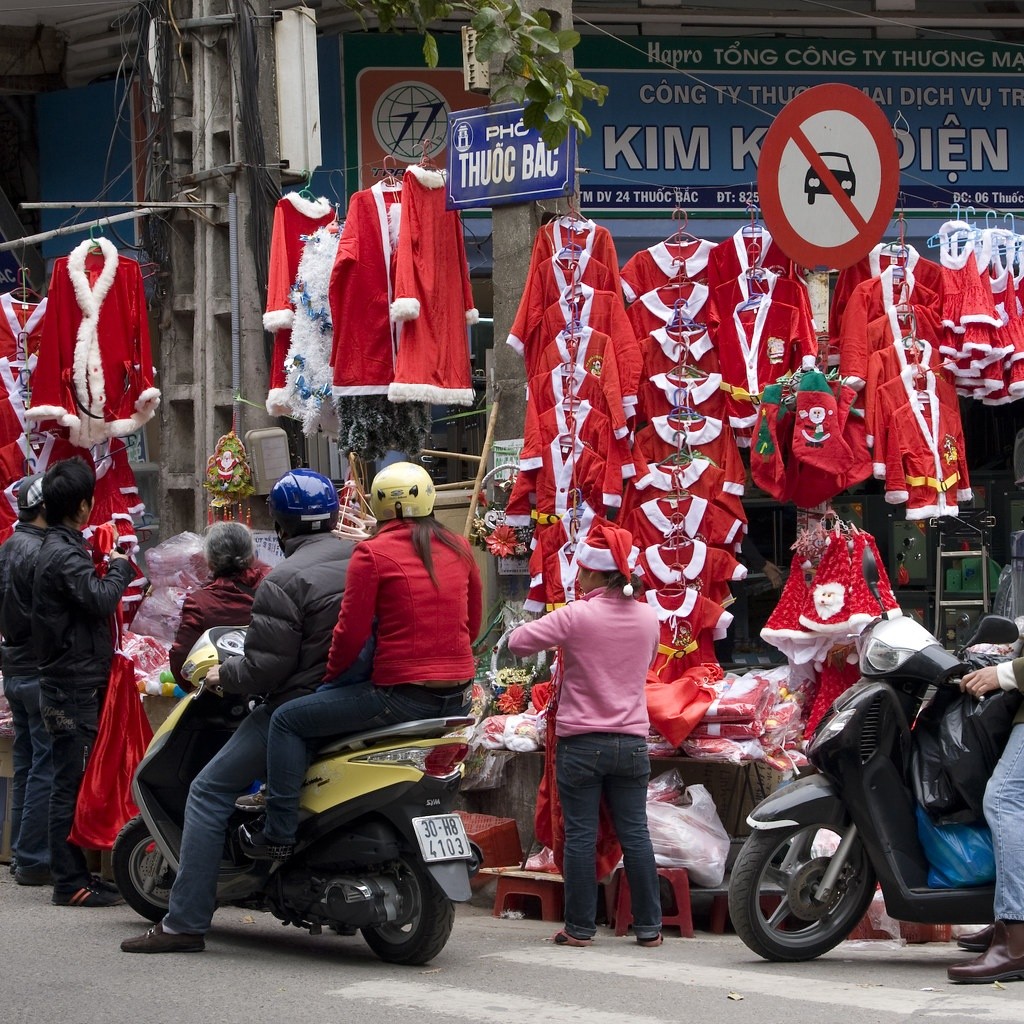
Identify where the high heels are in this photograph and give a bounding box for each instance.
[239,826,294,874]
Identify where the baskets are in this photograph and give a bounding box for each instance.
[473,465,535,544]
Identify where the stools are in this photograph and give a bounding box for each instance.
[611,867,697,939]
[493,878,564,923]
[709,895,786,936]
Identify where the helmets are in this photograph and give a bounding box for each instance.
[370,462,436,520]
[269,469,340,534]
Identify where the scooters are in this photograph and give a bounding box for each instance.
[110,624,484,969]
[726,539,1020,965]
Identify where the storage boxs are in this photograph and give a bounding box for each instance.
[451,810,525,887]
[847,887,951,944]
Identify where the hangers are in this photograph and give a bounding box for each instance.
[9,267,44,301]
[84,432,136,474]
[879,218,936,404]
[926,202,1024,262]
[413,138,441,172]
[558,191,589,556]
[297,169,323,203]
[380,156,404,188]
[129,513,156,543]
[660,204,773,594]
[790,497,869,556]
[81,224,104,257]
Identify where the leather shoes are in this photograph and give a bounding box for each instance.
[121,921,205,953]
[957,925,996,952]
[947,922,1024,983]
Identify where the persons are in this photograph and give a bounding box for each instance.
[31,456,137,906]
[168,521,274,694]
[508,526,664,946]
[119,469,375,953]
[0,472,64,887]
[238,461,482,873]
[947,620,1024,982]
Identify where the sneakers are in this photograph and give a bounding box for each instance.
[235,784,267,810]
[88,875,119,893]
[51,882,125,907]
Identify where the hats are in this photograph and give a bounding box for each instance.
[16,472,45,510]
[574,525,639,596]
[760,525,903,669]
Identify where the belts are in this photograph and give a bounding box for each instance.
[395,683,473,707]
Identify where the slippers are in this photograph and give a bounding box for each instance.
[554,929,592,946]
[637,932,663,947]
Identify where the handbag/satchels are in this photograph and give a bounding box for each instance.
[617,784,732,887]
[912,651,1022,887]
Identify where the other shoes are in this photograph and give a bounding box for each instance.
[14,866,47,885]
[9,857,18,874]
